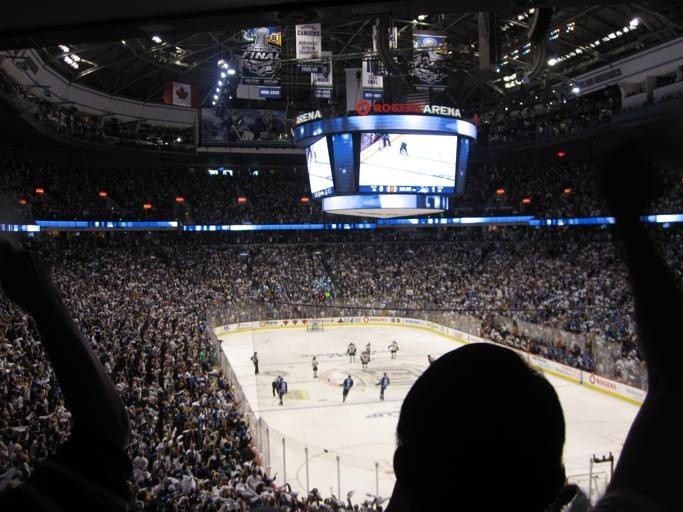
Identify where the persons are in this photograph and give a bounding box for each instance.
[199,109,284,142]
[1,153,683,512]
